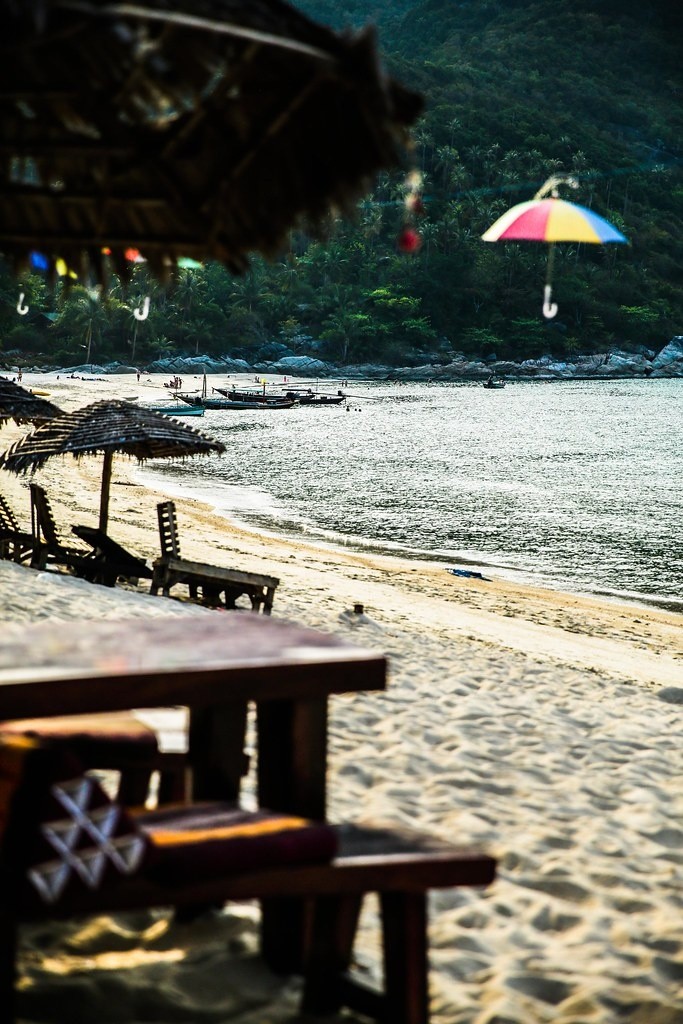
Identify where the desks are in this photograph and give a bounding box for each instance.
[0,611,391,971]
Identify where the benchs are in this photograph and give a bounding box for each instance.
[0,712,500,1024]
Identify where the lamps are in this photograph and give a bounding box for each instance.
[111,16,230,131]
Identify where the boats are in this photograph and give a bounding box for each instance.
[212,380,347,404]
[143,404,206,416]
[483,378,505,389]
[174,370,300,410]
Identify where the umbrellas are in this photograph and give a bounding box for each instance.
[0,0,423,290]
[480,198,630,320]
[0,399,226,536]
[16,247,204,321]
[0,376,67,429]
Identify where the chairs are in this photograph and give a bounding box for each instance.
[0,481,281,619]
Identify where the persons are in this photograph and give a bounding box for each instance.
[488,375,493,384]
[137,373,140,381]
[179,377,184,388]
